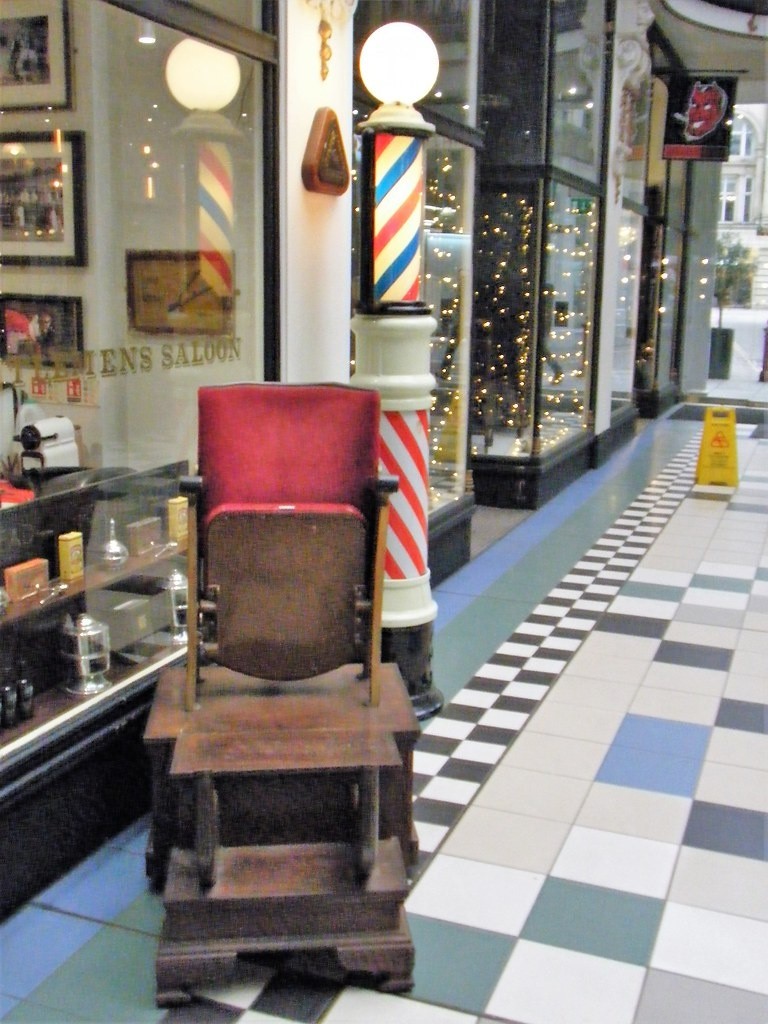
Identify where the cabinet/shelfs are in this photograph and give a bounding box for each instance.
[0,458,211,924]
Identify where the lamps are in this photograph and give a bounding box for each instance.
[350,19,440,722]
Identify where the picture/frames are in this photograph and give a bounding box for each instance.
[125,248,235,340]
[0,292,84,369]
[0,130,89,268]
[0,0,77,116]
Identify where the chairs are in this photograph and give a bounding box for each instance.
[177,382,402,713]
